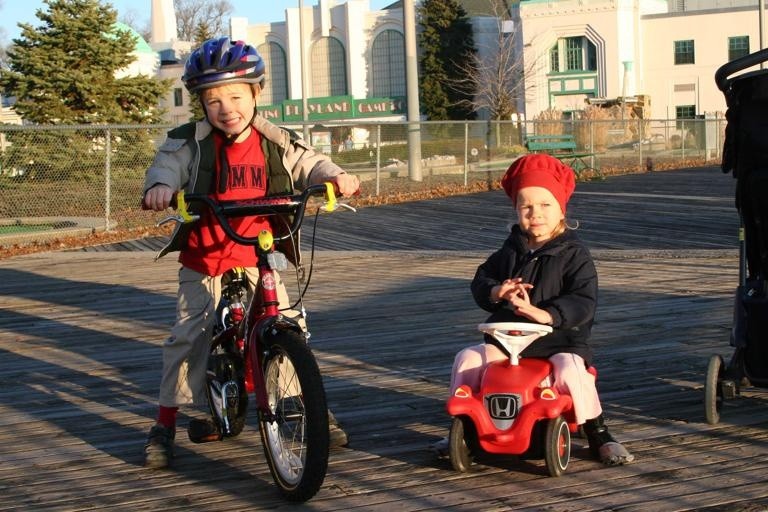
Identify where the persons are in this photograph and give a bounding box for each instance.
[139,34,362,470]
[431,151,636,468]
[330,135,373,153]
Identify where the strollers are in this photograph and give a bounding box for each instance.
[698,46,766,432]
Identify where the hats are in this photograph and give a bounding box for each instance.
[501,154,575,213]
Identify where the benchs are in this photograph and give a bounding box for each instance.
[527,135,576,154]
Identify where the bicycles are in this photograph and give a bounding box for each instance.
[140,174,364,506]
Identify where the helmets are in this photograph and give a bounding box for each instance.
[183,35,265,94]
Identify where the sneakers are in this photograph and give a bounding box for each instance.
[587,431,634,465]
[327,408,347,448]
[426,436,450,454]
[143,426,175,467]
